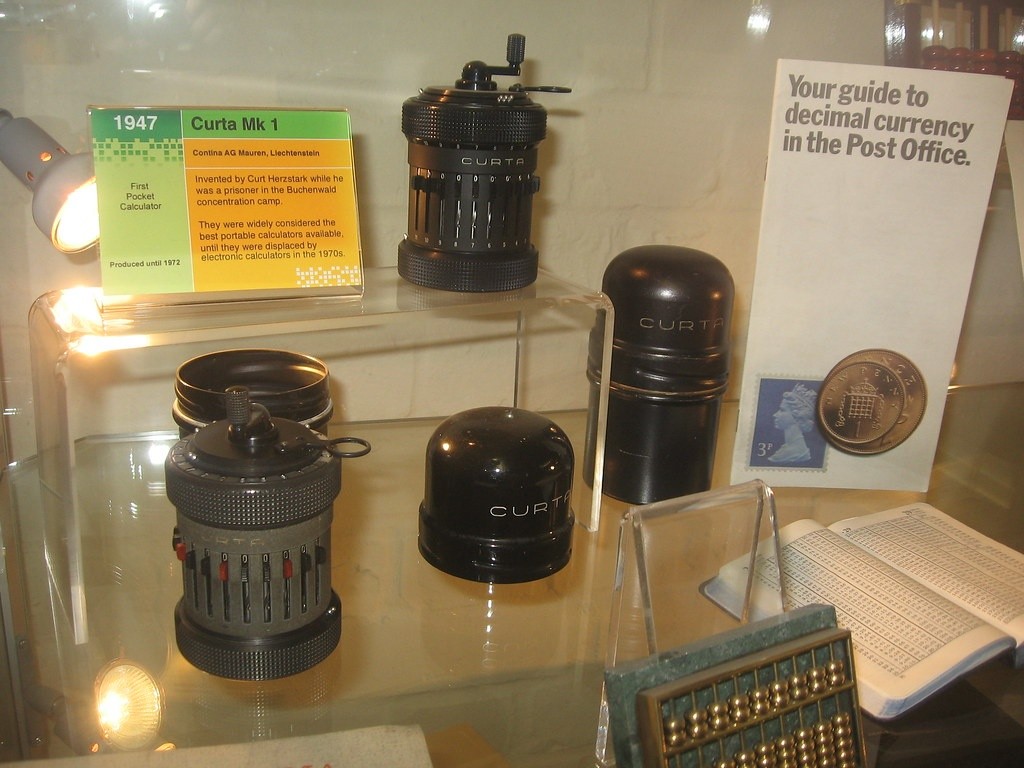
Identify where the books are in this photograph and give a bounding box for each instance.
[700,501,1024,724]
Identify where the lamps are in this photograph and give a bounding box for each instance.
[1,109,100,256]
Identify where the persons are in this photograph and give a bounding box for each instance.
[763,385,820,462]
